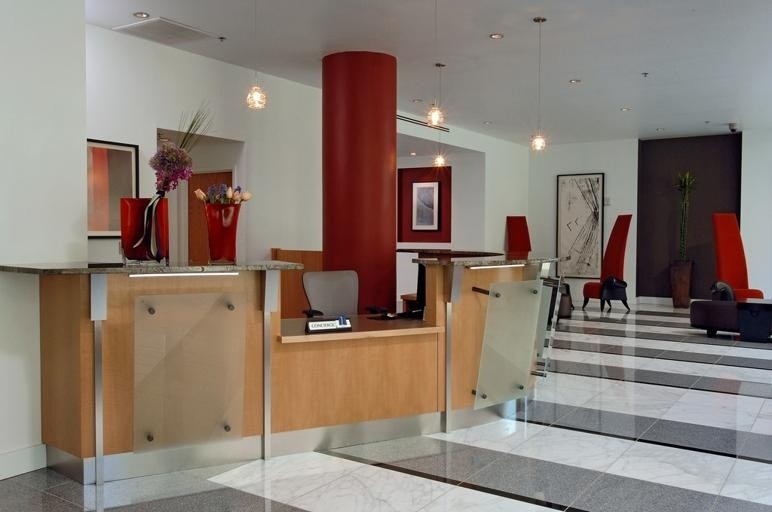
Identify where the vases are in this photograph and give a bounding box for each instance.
[119,195,170,262]
[556,172,604,280]
[205,203,240,264]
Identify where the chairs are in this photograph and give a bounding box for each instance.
[583,213,632,314]
[506,215,532,258]
[711,210,764,300]
[301,263,360,315]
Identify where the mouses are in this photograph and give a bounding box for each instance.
[385,312,396,318]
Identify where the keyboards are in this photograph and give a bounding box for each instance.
[397,310,423,319]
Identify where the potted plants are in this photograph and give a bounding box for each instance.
[668,171,695,307]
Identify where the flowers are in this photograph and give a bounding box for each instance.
[147,100,210,197]
[195,184,249,205]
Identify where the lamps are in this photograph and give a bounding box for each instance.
[428,2,443,127]
[247,3,268,109]
[436,146,444,166]
[533,18,547,150]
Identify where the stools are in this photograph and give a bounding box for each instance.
[691,297,772,343]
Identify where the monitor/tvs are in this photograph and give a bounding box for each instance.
[415,264,426,311]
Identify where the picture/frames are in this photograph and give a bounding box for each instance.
[412,182,438,231]
[89,137,141,239]
